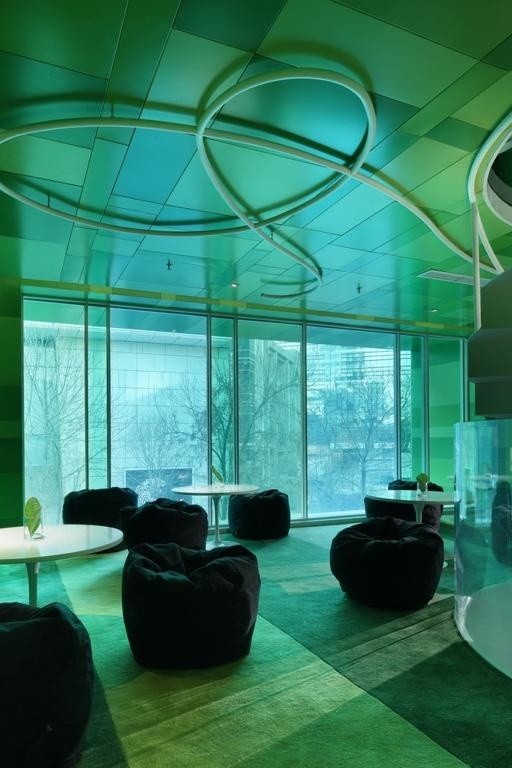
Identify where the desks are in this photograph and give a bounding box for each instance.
[366,490,457,569]
[173,482,261,551]
[0,522,125,609]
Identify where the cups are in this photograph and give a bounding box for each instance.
[23,515,47,541]
[416,481,428,498]
